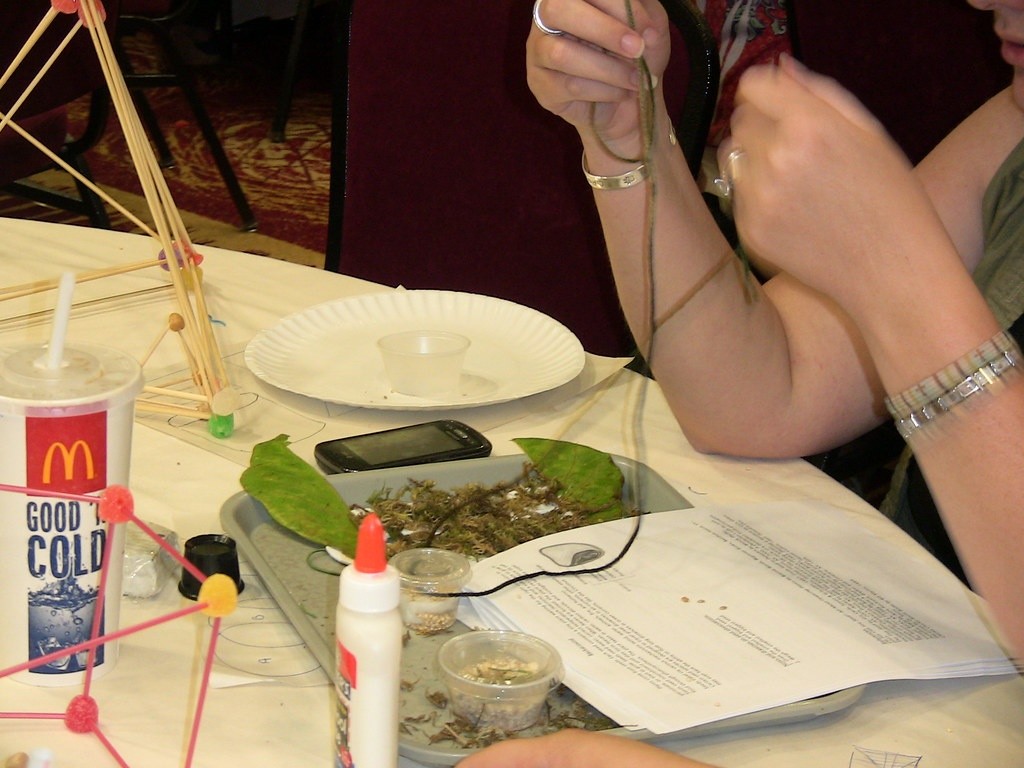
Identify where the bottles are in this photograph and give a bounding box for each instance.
[334,515,404,768]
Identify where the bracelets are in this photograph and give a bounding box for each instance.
[883,332,1021,437]
[582,113,677,188]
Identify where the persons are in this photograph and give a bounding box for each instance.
[454,728,714,768]
[526,0,1024,669]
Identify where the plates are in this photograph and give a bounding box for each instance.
[244,290,585,411]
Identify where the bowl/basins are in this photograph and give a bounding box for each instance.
[388,548,473,632]
[438,629,566,728]
[380,330,472,394]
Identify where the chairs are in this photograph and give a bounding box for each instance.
[0,0,1014,371]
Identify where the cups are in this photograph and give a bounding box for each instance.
[0,342,144,687]
[179,535,245,601]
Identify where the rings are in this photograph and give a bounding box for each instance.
[533,0,566,36]
[714,149,745,197]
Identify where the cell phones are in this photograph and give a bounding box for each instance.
[315,419,492,475]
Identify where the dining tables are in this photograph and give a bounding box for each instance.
[0,218,1024,768]
